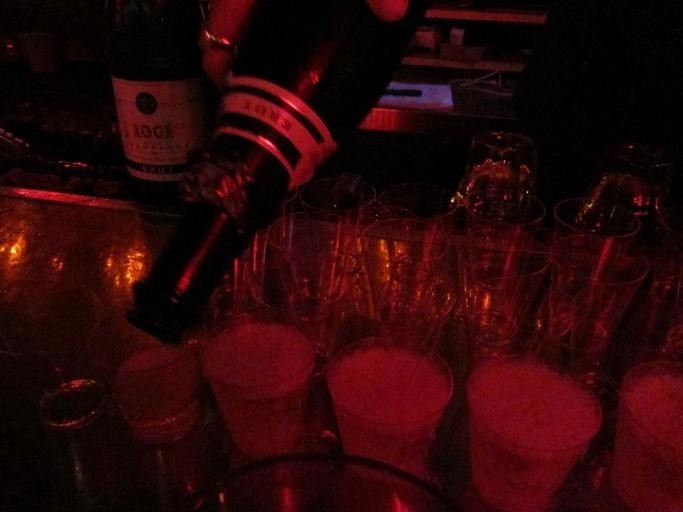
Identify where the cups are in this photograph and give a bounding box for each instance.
[0,129,681,510]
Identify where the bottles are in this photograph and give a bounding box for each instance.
[126,1,427,346]
[111,1,204,211]
